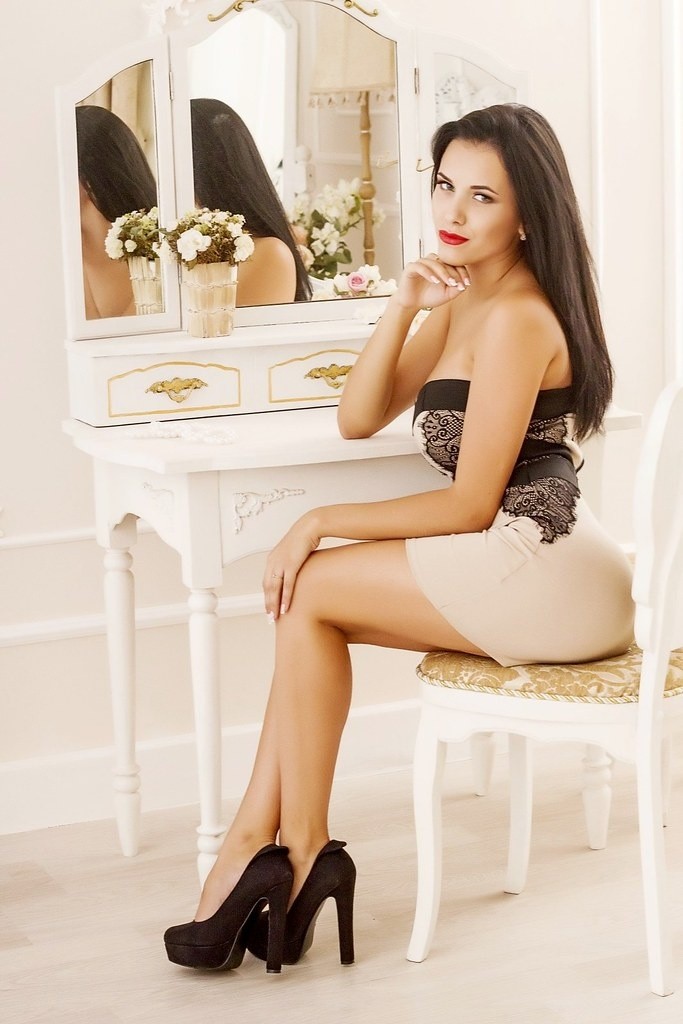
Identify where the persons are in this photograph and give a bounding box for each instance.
[73,104,165,324]
[165,104,634,976]
[184,99,315,308]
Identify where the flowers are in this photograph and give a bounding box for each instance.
[332,264,399,299]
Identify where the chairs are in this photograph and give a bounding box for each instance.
[403,374,683,998]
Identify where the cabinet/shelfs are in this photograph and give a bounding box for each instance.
[62,316,645,891]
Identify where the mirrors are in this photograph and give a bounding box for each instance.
[59,36,183,342]
[414,33,531,260]
[167,1,422,332]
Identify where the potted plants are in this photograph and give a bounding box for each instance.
[103,205,164,316]
[160,207,256,338]
[288,178,386,300]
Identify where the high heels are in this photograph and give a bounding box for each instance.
[249,841,358,966]
[164,844,293,974]
